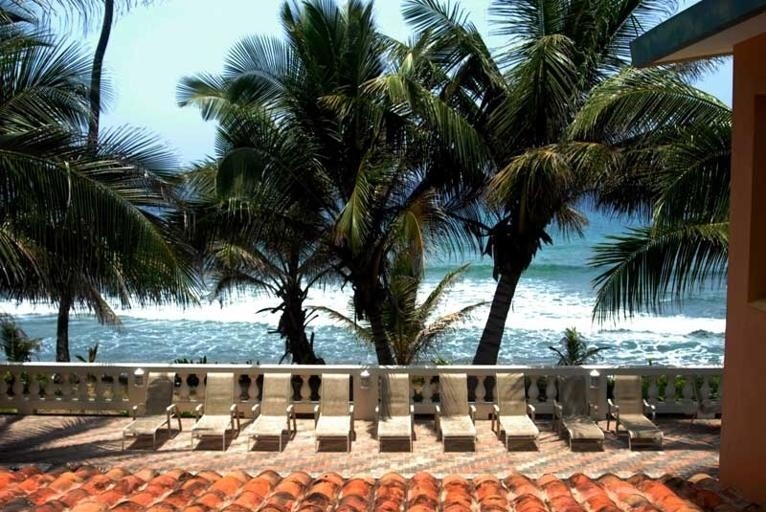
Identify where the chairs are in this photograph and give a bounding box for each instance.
[375,373,415,454]
[122,372,182,453]
[491,371,540,454]
[313,374,355,454]
[555,374,604,452]
[434,374,477,454]
[247,373,296,453]
[191,372,240,454]
[605,374,664,452]
